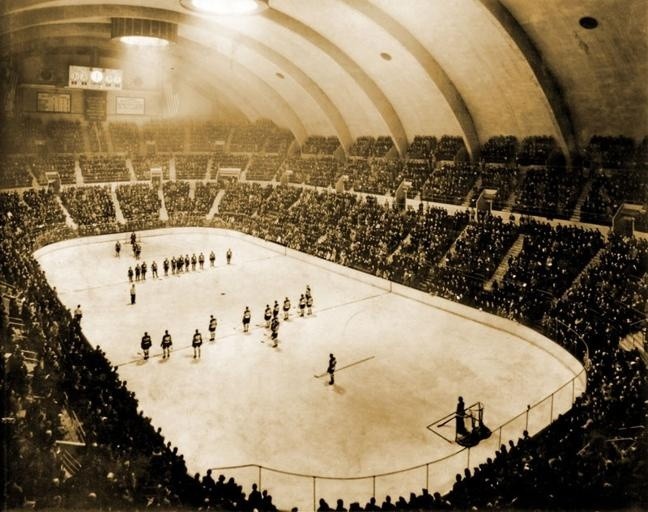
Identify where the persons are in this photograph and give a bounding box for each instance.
[327,352,338,385]
[262,284,313,348]
[140,331,152,360]
[447,258,648,511]
[160,329,172,359]
[130,282,137,305]
[0,261,120,511]
[120,378,446,511]
[207,314,218,341]
[192,328,202,359]
[242,306,251,332]
[2,112,648,295]
